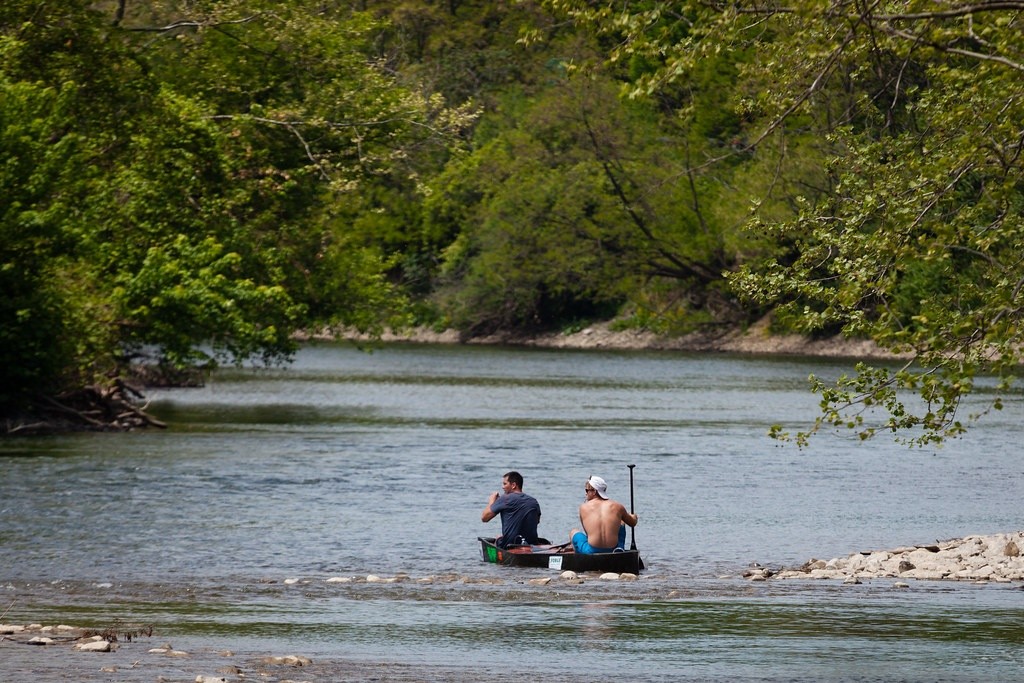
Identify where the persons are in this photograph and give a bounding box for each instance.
[569,476,637,554]
[481,471,541,547]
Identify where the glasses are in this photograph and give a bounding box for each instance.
[586,488,594,492]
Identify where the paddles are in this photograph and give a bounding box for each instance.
[626,464,636,549]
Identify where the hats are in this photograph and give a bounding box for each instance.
[588,475,609,499]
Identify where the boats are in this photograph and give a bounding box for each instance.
[478,536,641,575]
